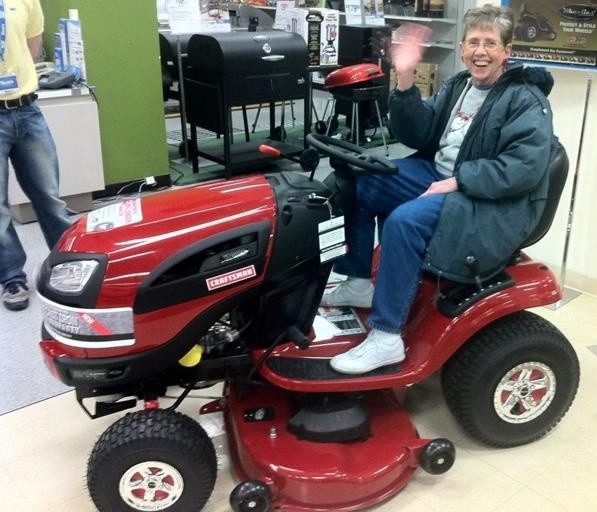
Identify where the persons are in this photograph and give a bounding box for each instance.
[0,0,74,311]
[316,2,555,374]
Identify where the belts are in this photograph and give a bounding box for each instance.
[0,91,39,111]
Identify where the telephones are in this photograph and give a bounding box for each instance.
[34,62,74,89]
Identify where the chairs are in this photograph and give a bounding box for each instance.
[377,137,572,275]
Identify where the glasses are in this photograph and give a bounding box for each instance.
[465,40,504,51]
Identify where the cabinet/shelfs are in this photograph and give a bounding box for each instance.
[383,12,457,50]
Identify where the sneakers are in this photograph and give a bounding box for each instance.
[319,280,376,309]
[329,327,407,374]
[2,281,29,311]
